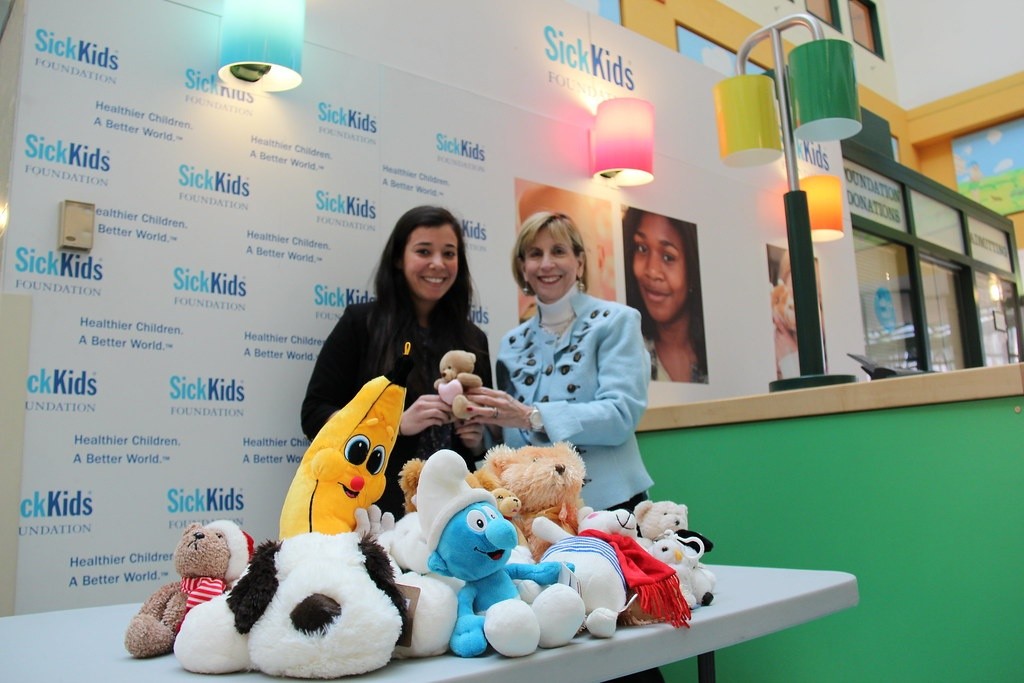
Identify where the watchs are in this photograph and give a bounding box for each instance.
[529,408,544,432]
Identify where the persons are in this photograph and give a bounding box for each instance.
[466,212,655,514]
[624,206,708,384]
[773,246,827,380]
[300,206,492,525]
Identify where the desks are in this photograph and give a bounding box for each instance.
[0,565,857,683]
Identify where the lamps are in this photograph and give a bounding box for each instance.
[590,98,656,187]
[800,174,845,242]
[714,72,781,167]
[218,0,309,94]
[788,38,862,143]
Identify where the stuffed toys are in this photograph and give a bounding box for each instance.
[125,350,716,680]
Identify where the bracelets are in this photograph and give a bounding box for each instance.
[399,425,403,436]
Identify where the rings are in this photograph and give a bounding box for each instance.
[494,406,498,418]
